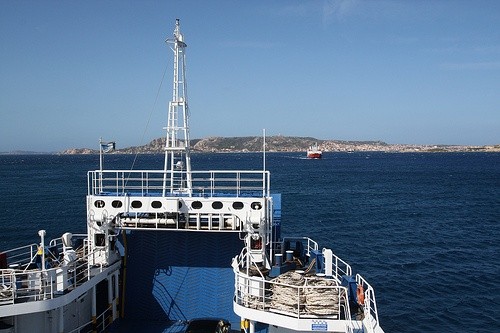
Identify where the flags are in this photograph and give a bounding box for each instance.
[99,139,116,155]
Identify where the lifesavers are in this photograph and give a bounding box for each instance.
[356,284,364,305]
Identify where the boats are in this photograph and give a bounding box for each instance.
[0,20,385,333]
[307,143,323,159]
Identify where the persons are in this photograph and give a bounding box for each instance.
[35,245,47,271]
